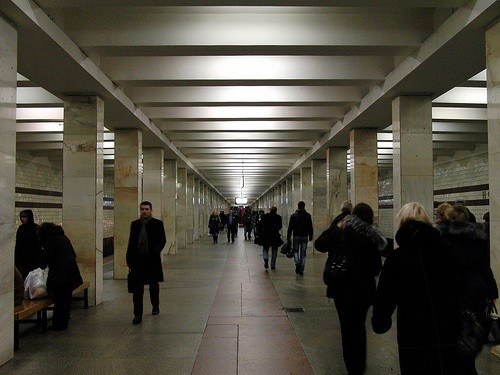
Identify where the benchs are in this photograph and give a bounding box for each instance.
[14,281,90,351]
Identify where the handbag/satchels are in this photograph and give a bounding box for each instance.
[281,242,292,255]
[26,267,48,300]
[488,303,500,345]
[127,266,134,294]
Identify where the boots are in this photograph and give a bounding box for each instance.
[213,235,218,244]
[244,233,252,240]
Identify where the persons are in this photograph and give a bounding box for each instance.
[38,223,82,332]
[126,201,166,325]
[14,210,41,306]
[208,206,285,269]
[315,202,387,375]
[372,202,500,375]
[287,202,313,276]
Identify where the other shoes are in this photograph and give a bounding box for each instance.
[47,324,66,331]
[295,263,303,275]
[264,259,269,268]
[132,314,142,325]
[152,303,159,315]
